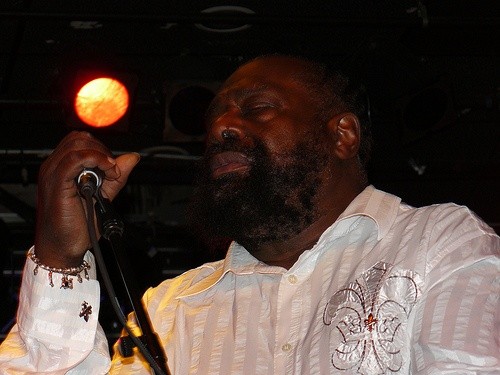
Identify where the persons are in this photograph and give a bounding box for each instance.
[1,46,500,375]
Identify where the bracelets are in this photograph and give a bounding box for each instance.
[28,255,91,289]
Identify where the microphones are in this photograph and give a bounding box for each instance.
[78,168,102,198]
